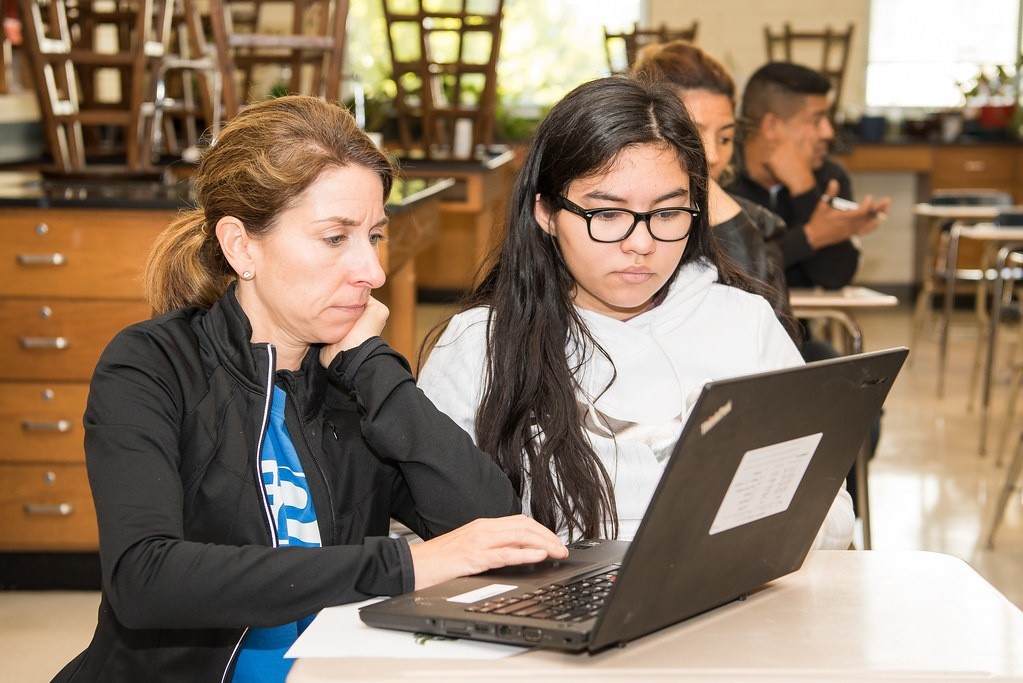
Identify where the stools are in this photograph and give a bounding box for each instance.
[1,0,1022,555]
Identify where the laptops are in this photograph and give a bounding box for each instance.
[358,345,911,652]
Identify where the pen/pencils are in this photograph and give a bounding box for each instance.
[822,192,888,220]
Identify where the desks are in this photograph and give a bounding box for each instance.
[1,137,1023,591]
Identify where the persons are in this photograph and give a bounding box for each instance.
[634,40,790,329]
[415,76,855,552]
[717,62,892,362]
[50,96,570,683]
